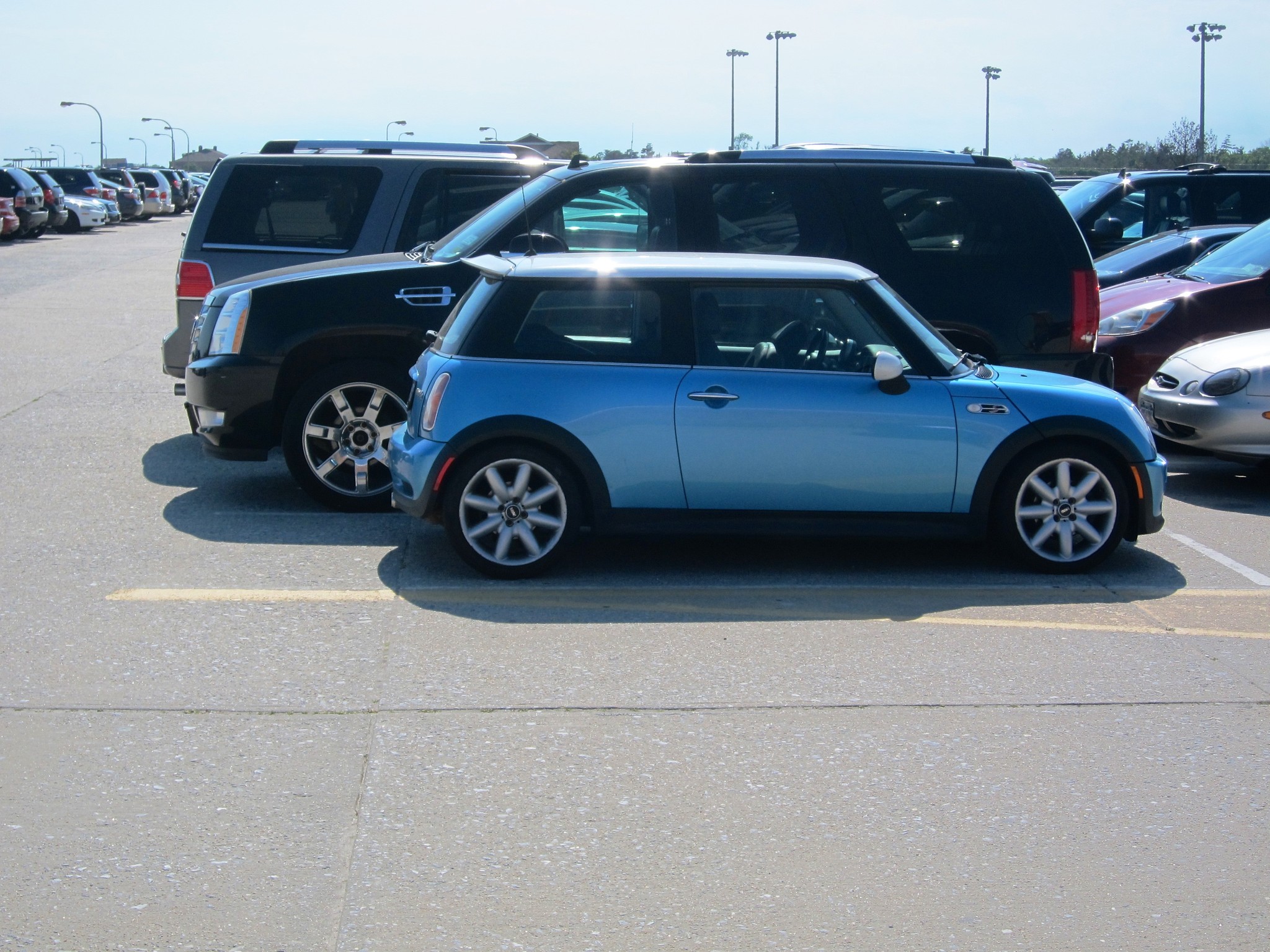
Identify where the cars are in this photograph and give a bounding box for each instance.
[1048,163,1268,464]
[387,251,1170,581]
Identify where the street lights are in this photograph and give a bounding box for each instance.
[142,118,174,169]
[164,127,189,173]
[25,133,176,171]
[766,30,797,147]
[60,101,103,169]
[727,49,749,149]
[1187,22,1226,166]
[981,66,1002,155]
[386,120,500,146]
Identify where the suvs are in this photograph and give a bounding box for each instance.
[0,164,212,242]
[180,143,1100,512]
[159,136,593,397]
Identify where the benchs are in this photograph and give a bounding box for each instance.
[517,324,598,363]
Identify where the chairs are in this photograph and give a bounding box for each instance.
[1150,193,1180,236]
[1173,199,1192,229]
[695,291,736,367]
